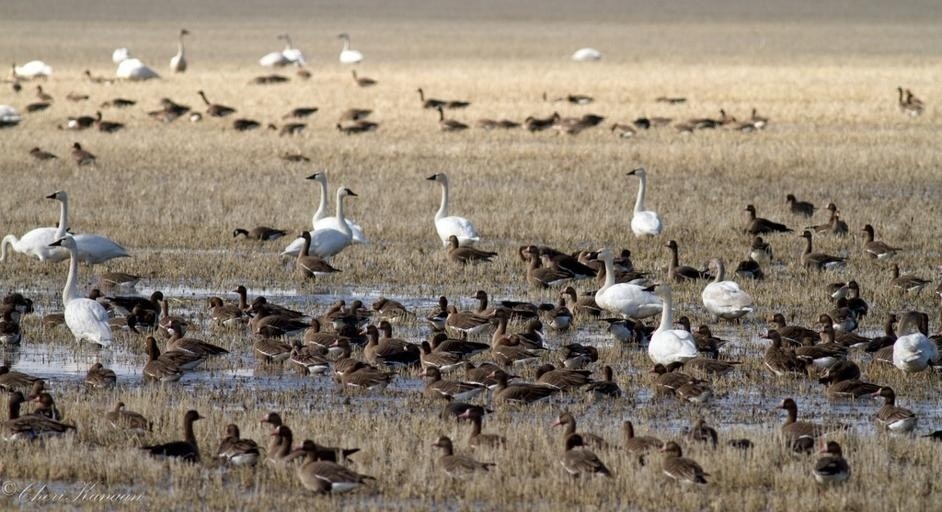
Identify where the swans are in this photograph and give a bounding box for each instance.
[427,172,482,246]
[568,46,602,65]
[306,168,368,247]
[637,280,699,367]
[278,33,303,62]
[168,28,189,73]
[335,30,361,66]
[885,307,936,374]
[277,184,359,261]
[46,188,130,269]
[48,236,115,356]
[117,59,160,81]
[258,50,288,67]
[113,48,132,60]
[698,254,755,323]
[0,228,74,273]
[10,59,51,79]
[625,167,660,238]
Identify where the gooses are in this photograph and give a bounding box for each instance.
[896,86,924,118]
[807,201,849,237]
[743,203,793,236]
[786,193,816,216]
[584,249,666,318]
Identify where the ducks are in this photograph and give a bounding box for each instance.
[0,224,942,495]
[0,69,769,163]
